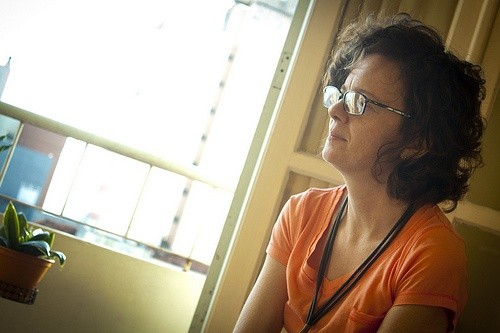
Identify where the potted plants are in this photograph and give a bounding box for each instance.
[0,199,66,288]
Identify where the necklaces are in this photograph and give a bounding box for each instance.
[298,194,420,333]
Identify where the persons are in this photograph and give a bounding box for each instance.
[233,12,487,333]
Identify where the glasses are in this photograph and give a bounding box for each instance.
[322,86,412,119]
